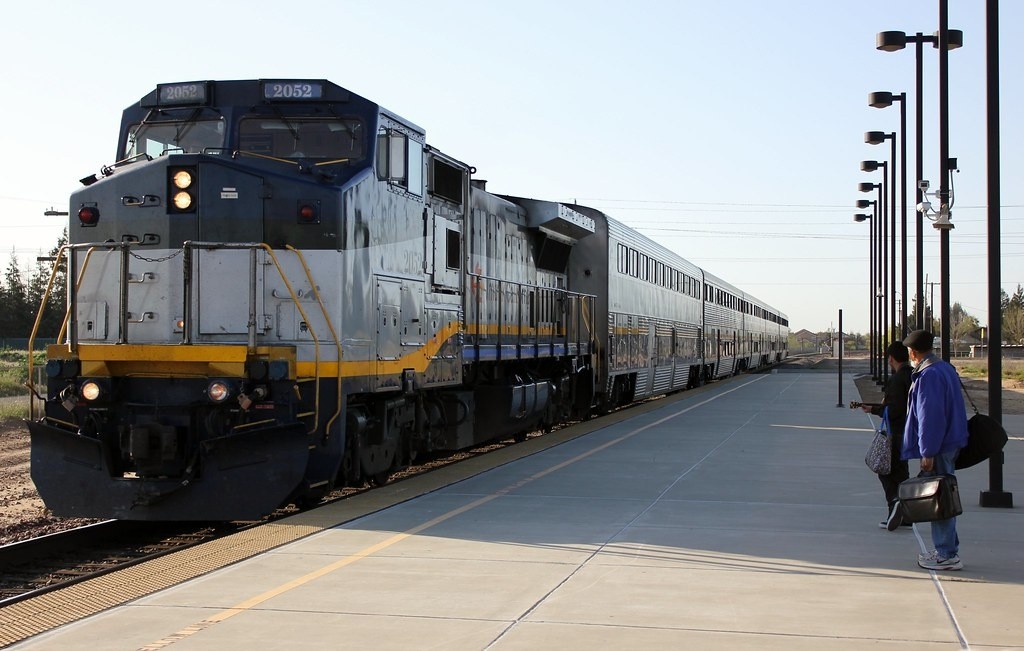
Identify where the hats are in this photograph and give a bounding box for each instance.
[902,329,934,353]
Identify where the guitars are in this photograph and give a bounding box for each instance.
[849,399,883,410]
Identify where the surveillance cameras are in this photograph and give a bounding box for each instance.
[918,201,931,212]
[918,180,930,191]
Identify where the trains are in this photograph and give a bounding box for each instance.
[22,75,791,524]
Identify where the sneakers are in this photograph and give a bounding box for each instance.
[879,520,913,529]
[887,498,901,531]
[918,548,963,570]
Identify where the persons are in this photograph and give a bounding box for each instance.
[898,329,968,571]
[860,340,915,532]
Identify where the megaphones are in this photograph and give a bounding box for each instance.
[932,214,955,229]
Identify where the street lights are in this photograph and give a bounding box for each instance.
[857,181,885,386]
[862,130,897,344]
[855,198,878,381]
[874,27,964,331]
[868,91,909,341]
[852,212,874,375]
[858,158,890,392]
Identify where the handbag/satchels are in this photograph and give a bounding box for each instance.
[898,467,962,523]
[954,414,1009,469]
[864,406,891,474]
